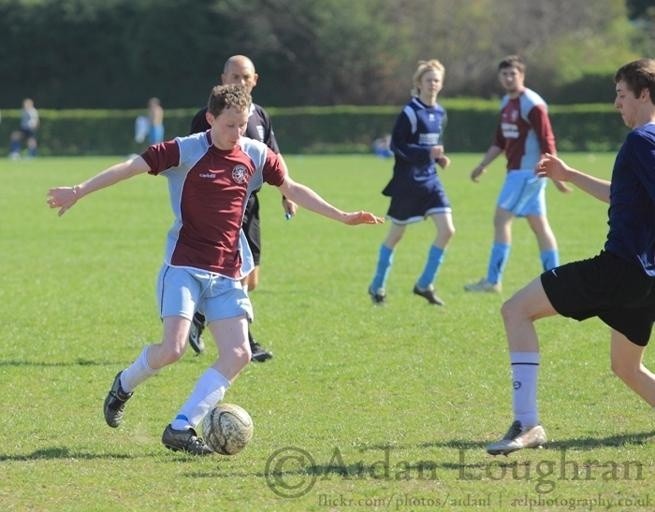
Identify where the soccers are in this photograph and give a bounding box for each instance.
[203,404,253,454]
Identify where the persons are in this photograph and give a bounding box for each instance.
[373,133,395,158]
[46,83,386,455]
[486,58,655,455]
[146,96,165,146]
[9,98,40,159]
[465,55,572,296]
[189,53,300,365]
[368,59,454,306]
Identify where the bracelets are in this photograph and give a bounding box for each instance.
[480,163,487,168]
[72,184,81,200]
[283,195,287,200]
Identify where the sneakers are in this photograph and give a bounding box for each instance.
[488,420,546,456]
[162,424,212,454]
[188,311,207,353]
[413,284,443,304]
[104,371,134,428]
[368,285,386,303]
[248,339,272,362]
[465,278,501,292]
